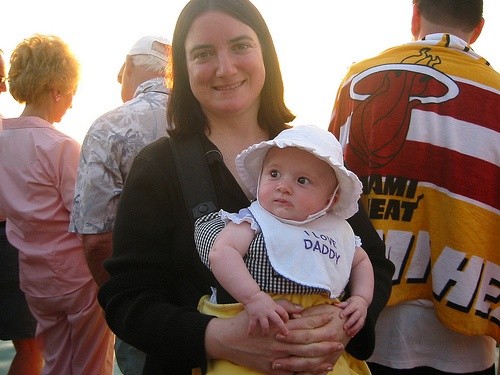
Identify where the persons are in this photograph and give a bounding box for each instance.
[97,1,396,375]
[193,124,374,375]
[0,48,46,375]
[65,36,173,375]
[0,33,117,375]
[327,0,500,375]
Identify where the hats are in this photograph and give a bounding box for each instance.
[234,124,363,224]
[117,35,172,84]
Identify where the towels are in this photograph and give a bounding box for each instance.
[196,294,373,375]
[328,32,500,347]
